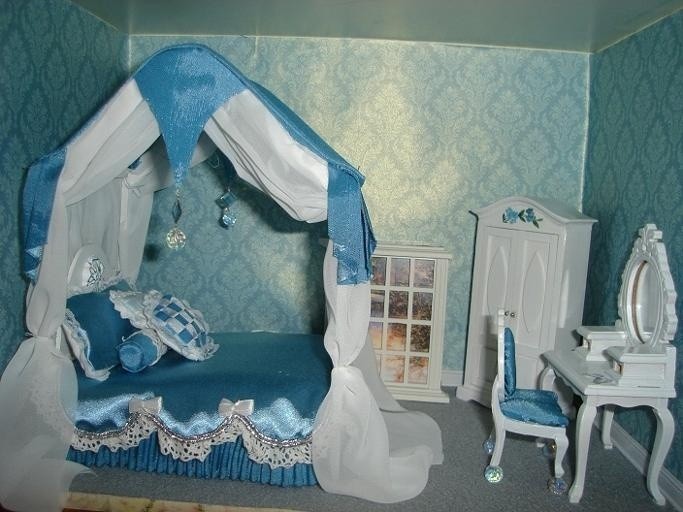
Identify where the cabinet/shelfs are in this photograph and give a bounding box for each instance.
[454,195,600,421]
[364,243,451,403]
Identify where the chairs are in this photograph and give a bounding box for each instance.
[483,308,569,496]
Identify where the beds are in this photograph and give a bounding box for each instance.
[0,44,444,512]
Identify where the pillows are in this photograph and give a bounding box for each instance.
[143,290,220,363]
[63,278,139,384]
[108,288,154,330]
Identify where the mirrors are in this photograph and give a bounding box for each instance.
[627,261,662,342]
